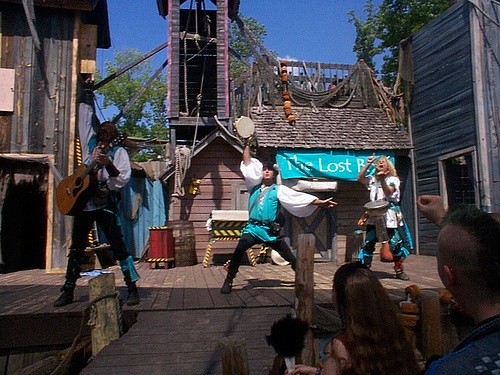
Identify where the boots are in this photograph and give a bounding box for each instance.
[119,256,140,306]
[54,249,85,306]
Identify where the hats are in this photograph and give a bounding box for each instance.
[263,160,278,175]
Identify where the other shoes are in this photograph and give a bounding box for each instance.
[394,266,409,280]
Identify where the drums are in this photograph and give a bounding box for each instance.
[361,199,390,217]
[233,116,254,139]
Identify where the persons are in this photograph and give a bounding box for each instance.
[418,195,500,375]
[358,153,414,281]
[220,132,338,294]
[54,77,140,307]
[284,263,419,375]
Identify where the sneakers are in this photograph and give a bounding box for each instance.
[220,279,232,294]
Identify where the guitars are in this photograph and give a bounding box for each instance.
[55,133,129,217]
